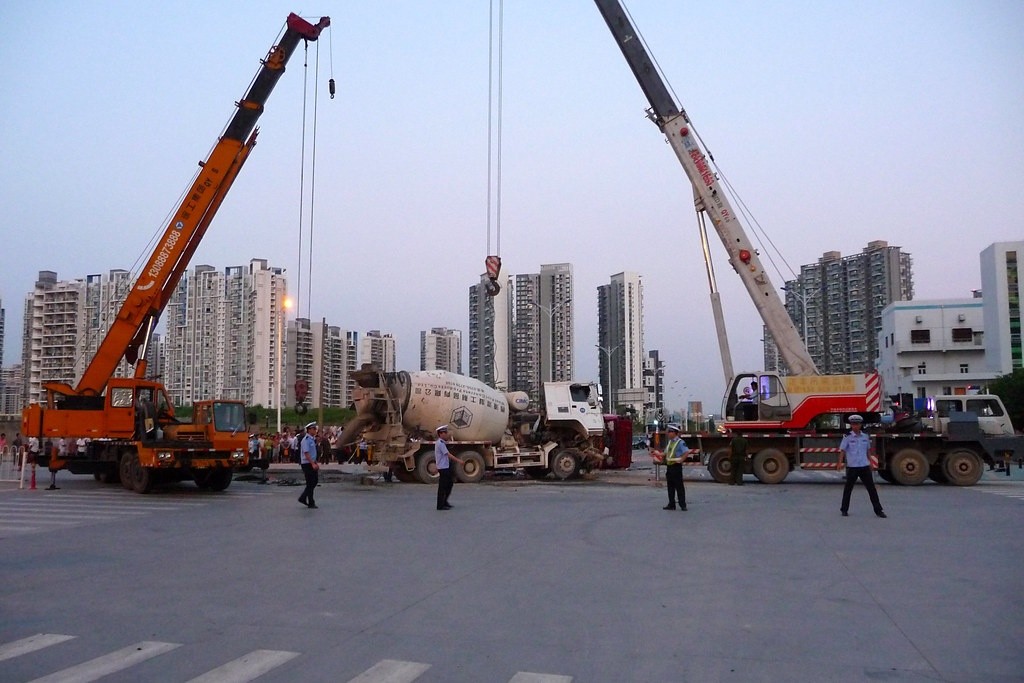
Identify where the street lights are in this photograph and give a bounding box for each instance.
[595,343,620,414]
[530,298,572,382]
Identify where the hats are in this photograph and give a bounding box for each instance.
[848,415,863,423]
[436,425,448,432]
[306,422,316,428]
[668,425,680,433]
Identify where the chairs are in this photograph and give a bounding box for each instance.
[743,385,767,419]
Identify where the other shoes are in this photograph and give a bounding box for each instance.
[308,504,317,509]
[876,510,886,518]
[682,506,687,511]
[663,505,676,510]
[841,511,848,516]
[299,496,308,505]
[437,502,452,509]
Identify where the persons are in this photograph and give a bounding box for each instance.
[29,437,40,455]
[0,432,7,452]
[76,438,91,455]
[12,434,22,464]
[58,437,68,456]
[837,415,887,518]
[298,421,320,508]
[248,425,305,464]
[739,381,758,420]
[654,424,690,510]
[314,430,366,465]
[435,424,465,509]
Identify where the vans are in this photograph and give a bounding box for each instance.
[920,395,1016,436]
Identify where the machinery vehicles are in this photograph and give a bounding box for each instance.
[333,360,609,483]
[485,0,1023,486]
[21,12,336,495]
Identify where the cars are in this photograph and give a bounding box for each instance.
[631,436,647,449]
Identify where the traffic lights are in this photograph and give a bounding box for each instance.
[889,393,913,417]
